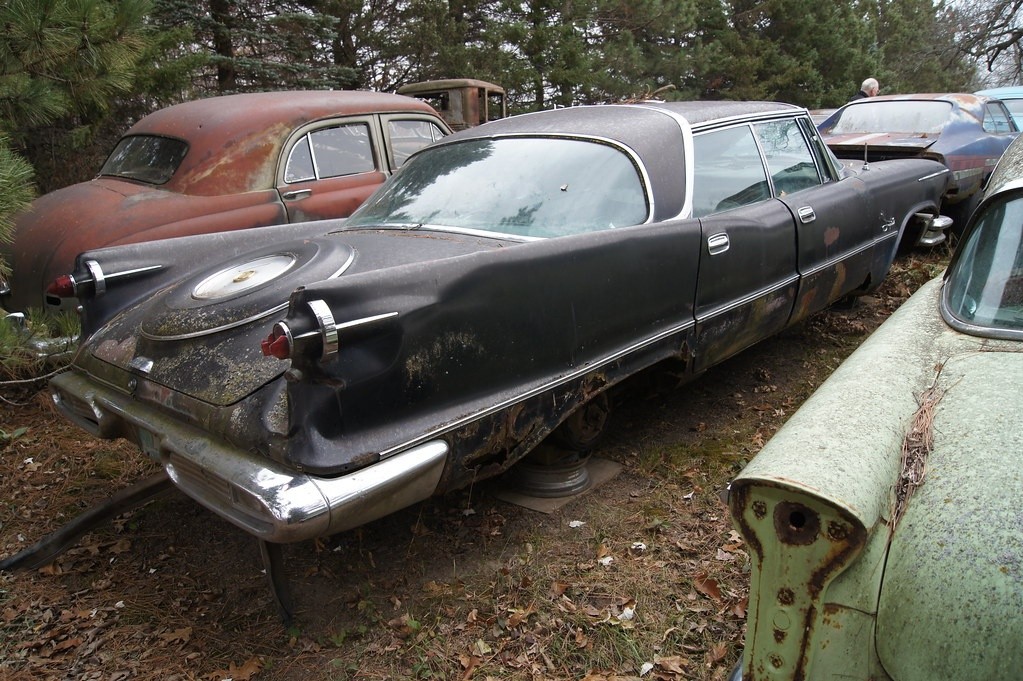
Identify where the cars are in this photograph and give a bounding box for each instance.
[52,101,954,549]
[725,125,1023,681]
[808,109,840,136]
[815,94,1023,237]
[1,89,456,361]
[971,86,1023,133]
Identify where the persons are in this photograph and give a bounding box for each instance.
[846,78,878,103]
